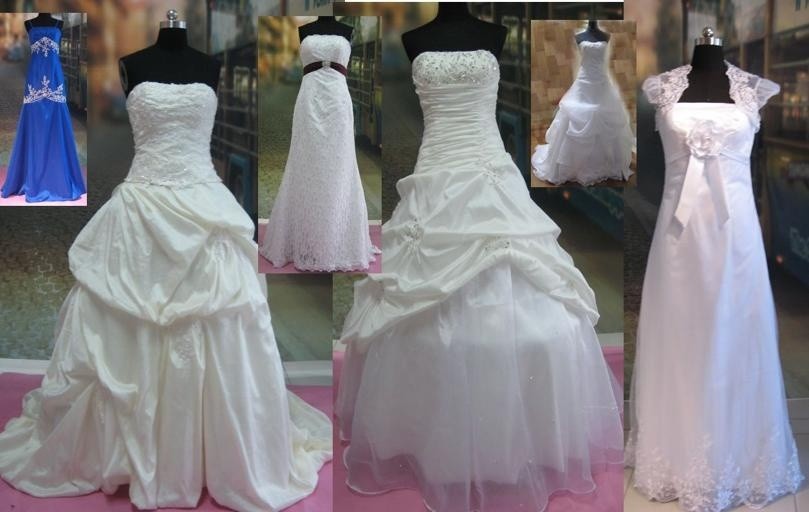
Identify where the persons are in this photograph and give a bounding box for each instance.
[332,3,626,512]
[0,13,88,203]
[531,20,637,187]
[0,10,334,511]
[257,16,382,273]
[627,27,808,509]
